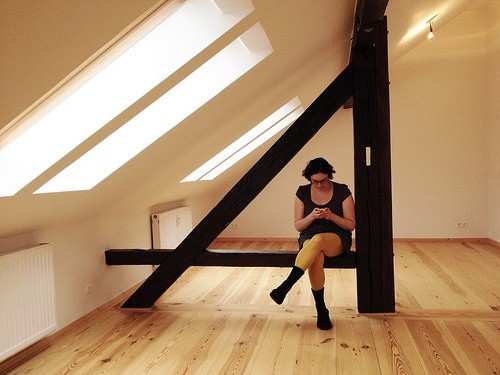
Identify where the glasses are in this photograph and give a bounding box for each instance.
[311,177,329,184]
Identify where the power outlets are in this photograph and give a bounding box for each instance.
[457,222,467,227]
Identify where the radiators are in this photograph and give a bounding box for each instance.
[151,205,191,269]
[0,243,57,363]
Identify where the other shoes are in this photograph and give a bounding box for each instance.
[316,309,333,330]
[269,285,286,305]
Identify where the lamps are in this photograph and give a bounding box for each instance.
[428,22,435,39]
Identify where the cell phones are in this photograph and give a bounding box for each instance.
[318,209,325,212]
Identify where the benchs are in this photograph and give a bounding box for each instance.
[105,249,356,308]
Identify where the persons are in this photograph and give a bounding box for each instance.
[270,157,355,331]
